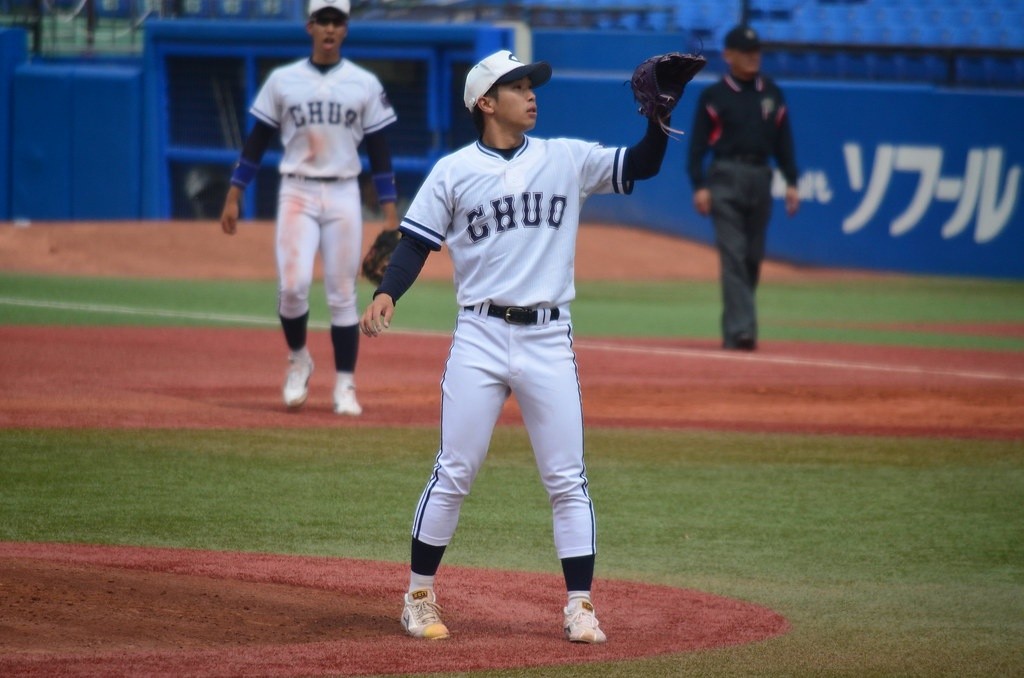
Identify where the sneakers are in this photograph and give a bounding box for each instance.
[563,598,606,643]
[284,350,314,407]
[401,589,450,640]
[333,380,361,415]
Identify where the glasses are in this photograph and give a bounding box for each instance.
[310,17,346,27]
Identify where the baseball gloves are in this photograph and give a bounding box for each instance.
[363,230,402,282]
[629,50,708,124]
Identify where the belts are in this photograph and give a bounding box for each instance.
[288,174,338,182]
[714,151,768,165]
[464,303,559,325]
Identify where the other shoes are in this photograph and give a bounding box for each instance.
[723,334,754,351]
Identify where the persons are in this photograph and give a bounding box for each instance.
[360,51,707,641]
[687,24,799,351]
[221,0,398,415]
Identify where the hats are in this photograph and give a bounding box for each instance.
[308,0,350,16]
[464,50,552,114]
[725,27,763,52]
